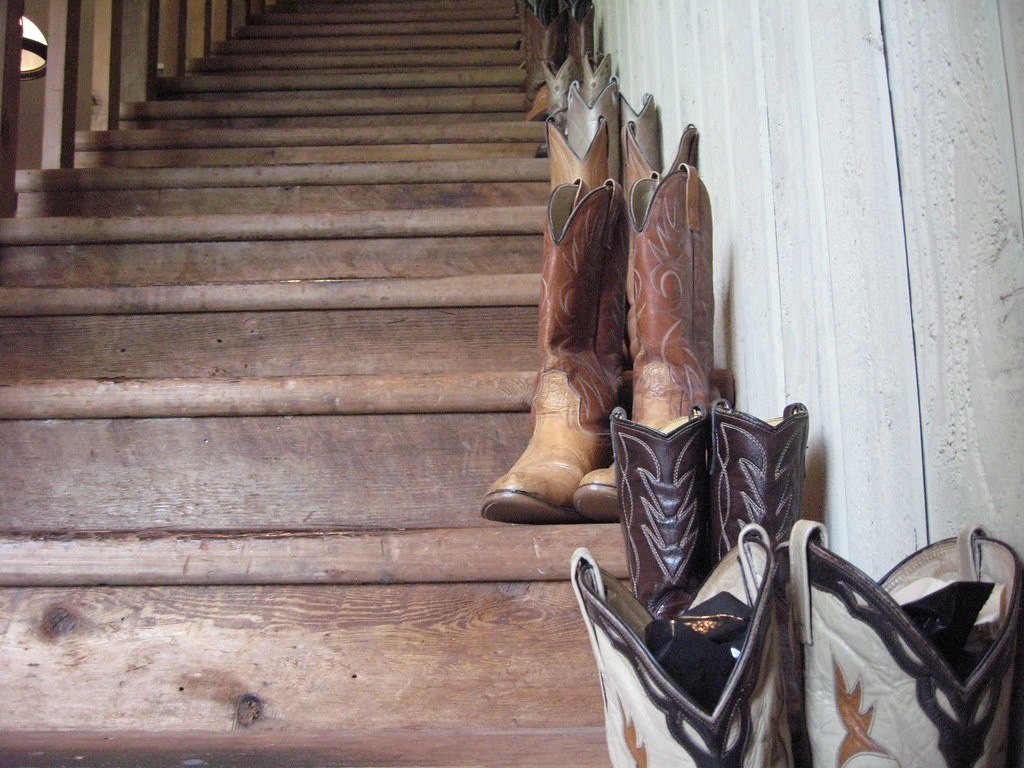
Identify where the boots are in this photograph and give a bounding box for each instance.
[515,0,611,158]
[609,406,708,624]
[574,164,714,521]
[705,399,810,584]
[619,92,664,176]
[482,176,626,526]
[778,518,1023,768]
[564,76,620,182]
[570,525,792,768]
[549,116,612,200]
[624,120,699,225]
